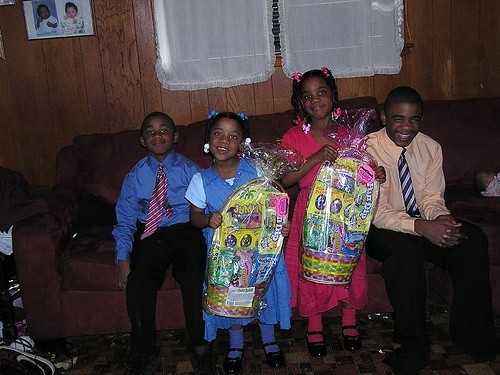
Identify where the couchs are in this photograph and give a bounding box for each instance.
[14,96,500,352]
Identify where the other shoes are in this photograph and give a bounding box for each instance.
[305,330,328,357]
[223,346,244,373]
[342,325,362,351]
[262,340,286,369]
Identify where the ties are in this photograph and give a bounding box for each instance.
[397,147,422,218]
[140,162,174,240]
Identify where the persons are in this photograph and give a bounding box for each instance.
[60,1,85,35]
[111,111,217,375]
[183,110,291,375]
[355,86,497,375]
[34,4,60,36]
[273,67,386,357]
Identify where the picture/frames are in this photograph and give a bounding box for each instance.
[21,0,96,41]
